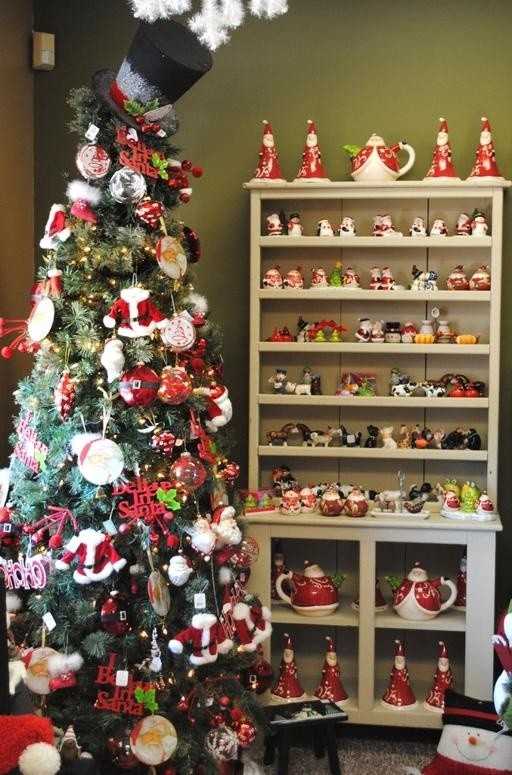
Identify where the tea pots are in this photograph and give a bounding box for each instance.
[274,559,348,617]
[384,561,459,622]
[341,132,417,183]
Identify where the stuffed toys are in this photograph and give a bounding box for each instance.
[491,599,512,734]
[400,688,512,775]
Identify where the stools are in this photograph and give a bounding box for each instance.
[263,699,348,775]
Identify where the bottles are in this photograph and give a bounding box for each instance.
[384,321,416,342]
[444,263,490,290]
[419,320,451,334]
[280,486,369,517]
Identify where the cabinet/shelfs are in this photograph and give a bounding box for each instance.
[257,187,493,450]
[270,524,467,722]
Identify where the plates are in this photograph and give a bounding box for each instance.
[370,506,430,521]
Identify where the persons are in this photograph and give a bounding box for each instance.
[0,117,512,775]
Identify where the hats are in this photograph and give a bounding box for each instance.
[91,18,213,139]
